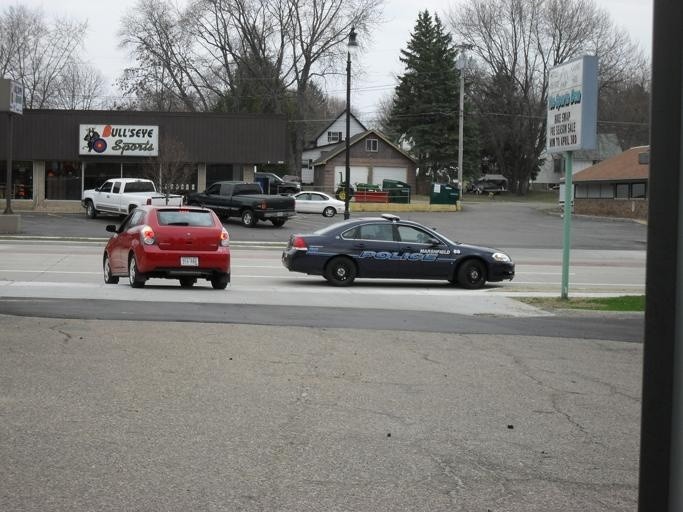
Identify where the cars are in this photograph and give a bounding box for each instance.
[287,190,346,218]
[470,175,508,195]
[100,205,231,291]
[279,214,515,291]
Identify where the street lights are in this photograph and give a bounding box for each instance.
[453,41,474,201]
[342,25,358,222]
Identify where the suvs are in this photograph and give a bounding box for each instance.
[255,172,303,196]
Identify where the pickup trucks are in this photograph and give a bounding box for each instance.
[187,181,296,228]
[81,176,185,220]
